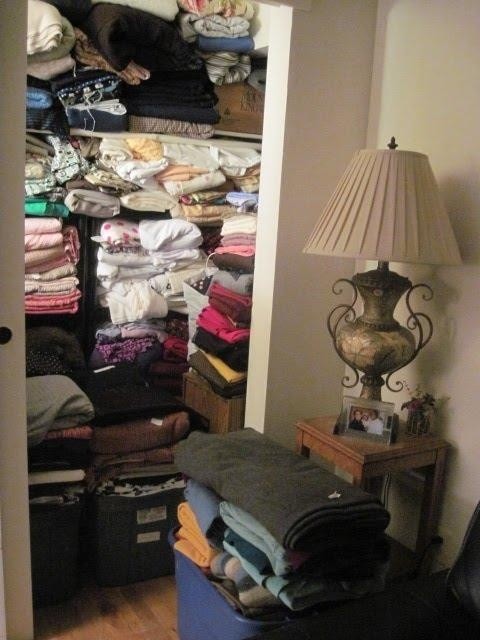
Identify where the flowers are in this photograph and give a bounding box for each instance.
[400,380,453,427]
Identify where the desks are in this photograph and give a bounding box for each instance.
[293,407,452,575]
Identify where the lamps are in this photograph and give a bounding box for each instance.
[301,137,465,401]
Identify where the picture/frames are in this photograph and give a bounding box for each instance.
[333,395,397,444]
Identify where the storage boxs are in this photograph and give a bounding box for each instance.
[169,525,415,640]
[91,471,186,587]
[29,500,87,609]
[182,371,245,436]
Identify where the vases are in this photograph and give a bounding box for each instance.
[405,408,430,436]
